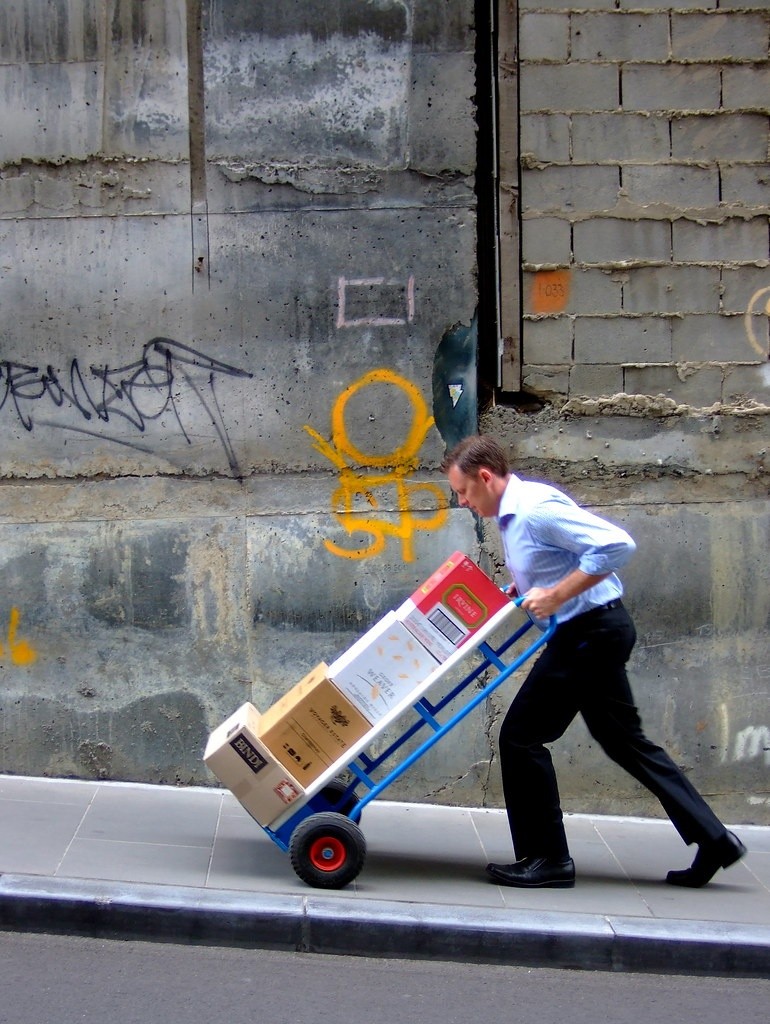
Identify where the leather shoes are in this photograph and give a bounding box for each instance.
[665,831,747,887]
[485,850,575,887]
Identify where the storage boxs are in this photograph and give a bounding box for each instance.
[397,552,513,665]
[204,701,305,829]
[325,610,441,727]
[258,661,371,789]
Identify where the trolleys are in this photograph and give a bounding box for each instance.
[259,584,560,890]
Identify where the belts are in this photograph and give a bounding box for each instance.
[599,599,622,611]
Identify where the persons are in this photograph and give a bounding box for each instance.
[445,436,746,887]
